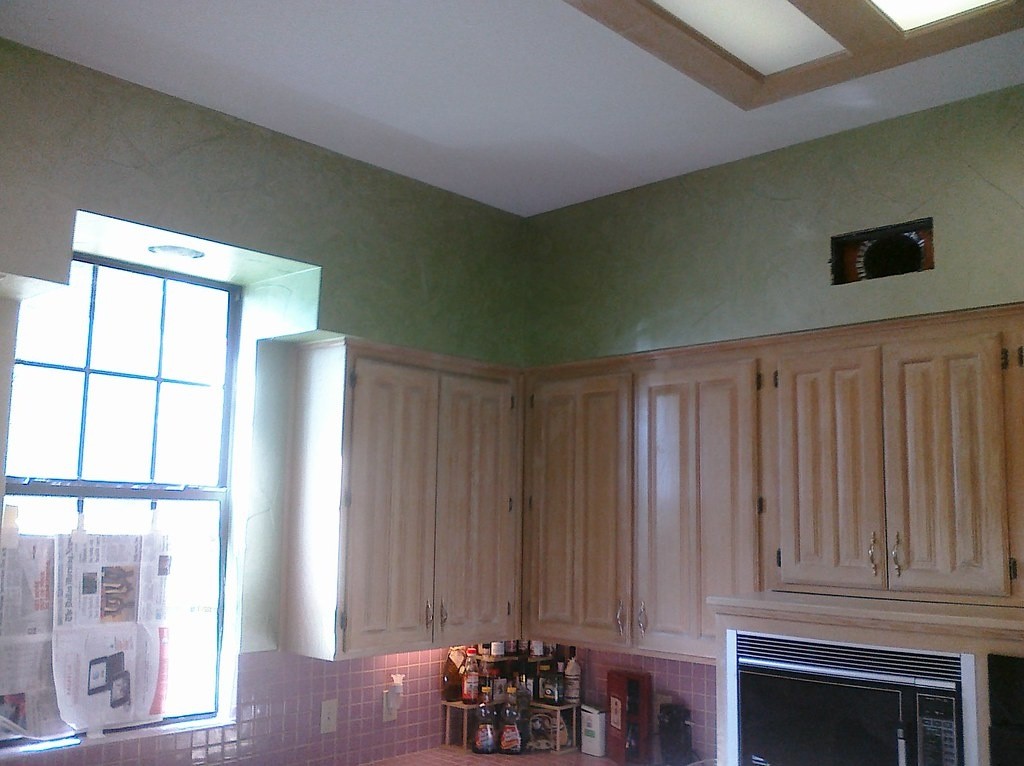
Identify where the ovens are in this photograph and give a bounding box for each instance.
[723,629,980,766]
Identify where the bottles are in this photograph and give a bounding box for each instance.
[442,640,580,753]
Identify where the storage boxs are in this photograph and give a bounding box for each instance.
[580,703,607,758]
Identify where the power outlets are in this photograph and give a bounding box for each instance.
[383,689,398,723]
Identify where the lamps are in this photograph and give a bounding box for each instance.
[388,673,406,715]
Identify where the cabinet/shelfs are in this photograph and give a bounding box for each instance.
[521,340,757,668]
[280,337,526,661]
[758,301,1011,661]
